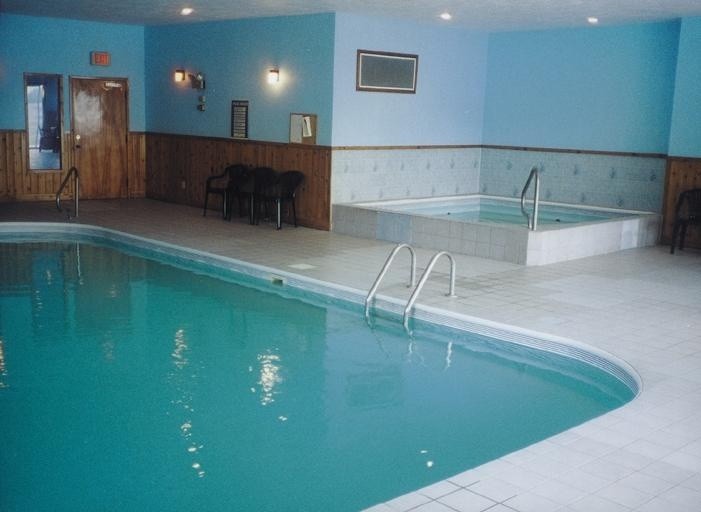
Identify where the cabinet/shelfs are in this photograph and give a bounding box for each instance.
[38,111,59,154]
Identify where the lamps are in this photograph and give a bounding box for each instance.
[174,69,186,82]
[269,68,281,85]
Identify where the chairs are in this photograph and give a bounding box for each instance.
[202,164,306,231]
[671,188,701,255]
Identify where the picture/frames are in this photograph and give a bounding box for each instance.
[356,49,420,95]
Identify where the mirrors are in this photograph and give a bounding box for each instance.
[22,71,66,174]
